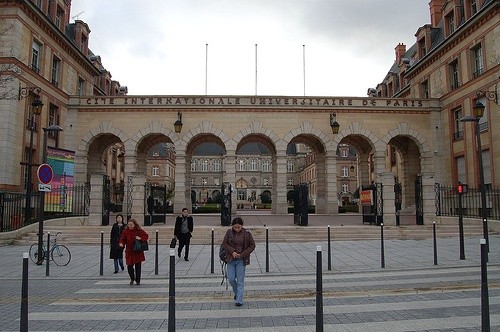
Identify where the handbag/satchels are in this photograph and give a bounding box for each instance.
[133,240,149,251]
[170,237,177,248]
[218,242,227,263]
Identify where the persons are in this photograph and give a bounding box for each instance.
[241,203,244,209]
[109,214,125,274]
[119,220,150,287]
[237,204,240,209]
[222,218,256,307]
[173,208,194,261]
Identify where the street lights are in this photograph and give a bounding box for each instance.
[458,116,490,253]
[37,124,63,265]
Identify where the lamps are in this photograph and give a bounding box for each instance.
[20,86,44,114]
[118,154,123,162]
[174,111,183,133]
[350,161,354,172]
[330,112,340,134]
[472,84,497,117]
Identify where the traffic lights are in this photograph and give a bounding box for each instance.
[457,185,468,195]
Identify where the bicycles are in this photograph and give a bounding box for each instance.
[29,232,71,266]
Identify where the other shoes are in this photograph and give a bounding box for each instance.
[234,295,236,300]
[121,266,124,271]
[236,303,242,306]
[129,280,134,286]
[184,257,189,261]
[114,270,119,274]
[137,282,140,285]
[178,252,181,258]
